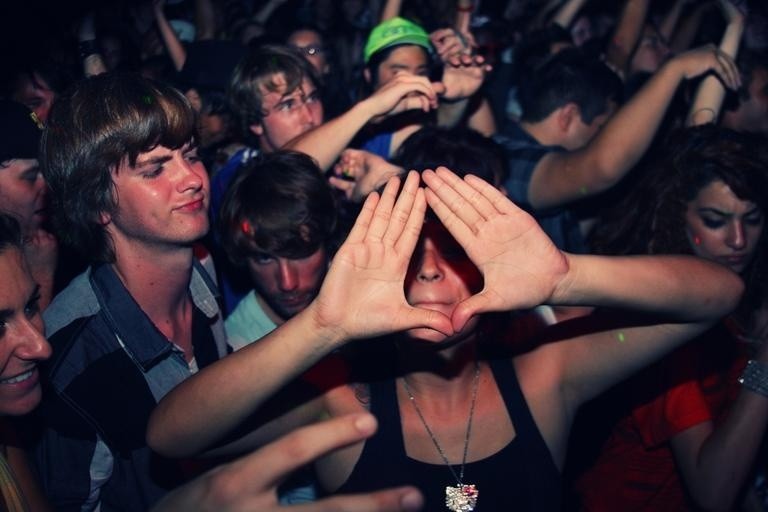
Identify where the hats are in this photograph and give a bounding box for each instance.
[362,15,437,68]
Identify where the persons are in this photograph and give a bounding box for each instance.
[2,0,768,511]
